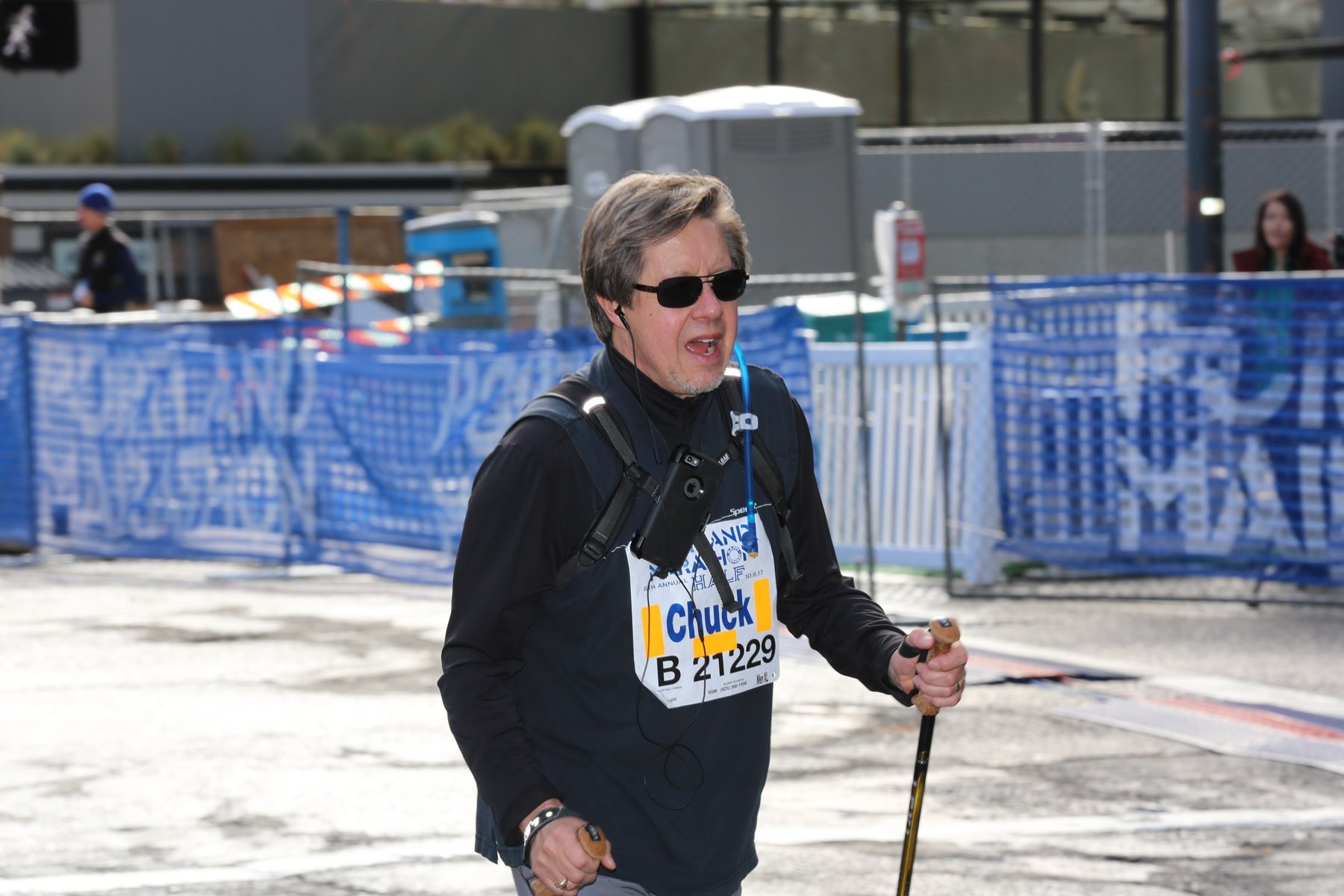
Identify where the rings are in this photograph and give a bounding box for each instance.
[555,878,568,890]
[956,682,961,692]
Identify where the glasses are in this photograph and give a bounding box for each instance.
[631,268,749,309]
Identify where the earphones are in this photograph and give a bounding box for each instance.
[614,303,631,331]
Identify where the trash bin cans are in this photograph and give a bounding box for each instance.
[404,208,510,330]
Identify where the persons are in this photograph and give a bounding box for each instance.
[70,181,149,314]
[438,174,968,896]
[1230,192,1344,587]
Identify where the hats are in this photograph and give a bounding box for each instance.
[78,183,116,215]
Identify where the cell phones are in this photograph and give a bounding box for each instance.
[632,446,726,575]
